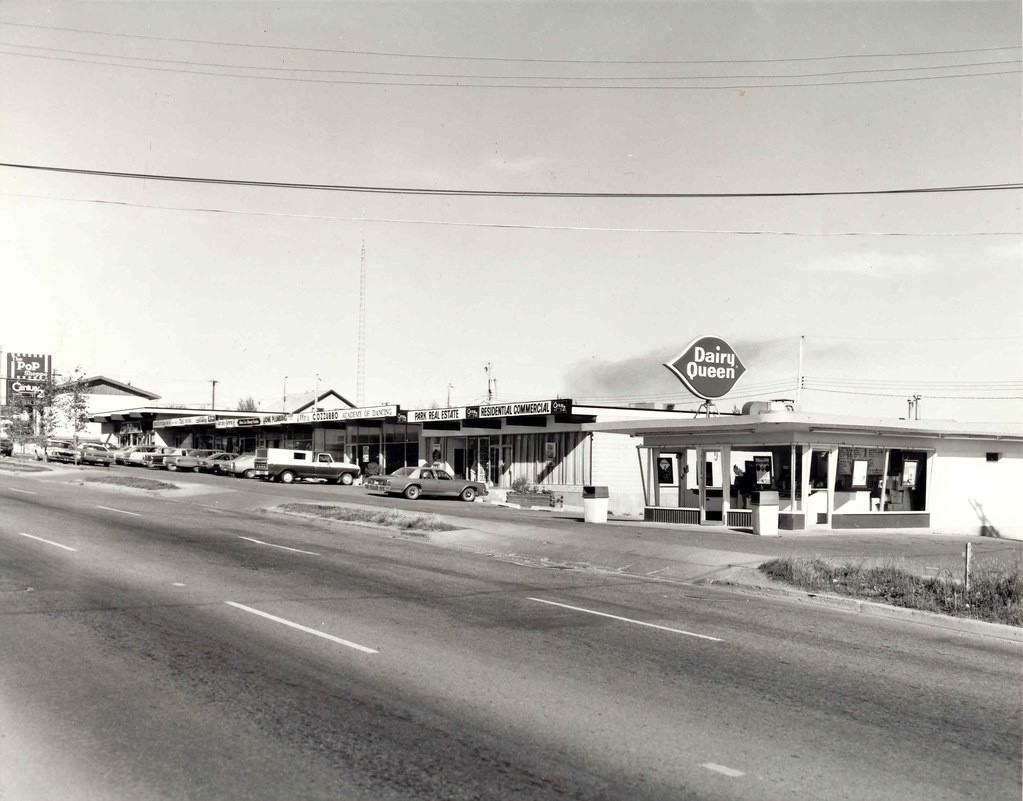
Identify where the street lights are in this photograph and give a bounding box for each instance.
[211,380,218,409]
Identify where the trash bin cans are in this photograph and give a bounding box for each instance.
[582,485,610,523]
[750,491,780,536]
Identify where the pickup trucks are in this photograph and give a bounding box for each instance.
[253,448,360,485]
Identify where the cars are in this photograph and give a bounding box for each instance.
[33,439,256,479]
[365,467,490,502]
[0,432,14,457]
